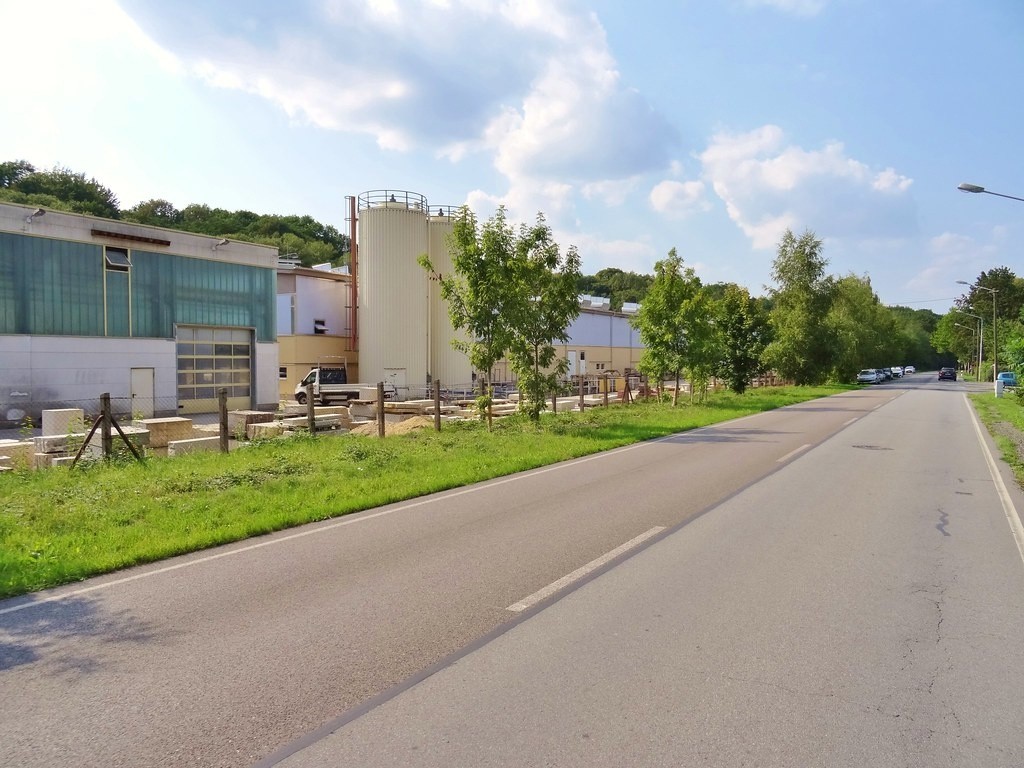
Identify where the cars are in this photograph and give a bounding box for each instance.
[938,367,958,381]
[875,369,886,380]
[996,371,1019,389]
[891,367,903,378]
[857,369,882,384]
[883,368,892,379]
[900,366,905,375]
[904,366,915,374]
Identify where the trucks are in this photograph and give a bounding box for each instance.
[294,369,370,406]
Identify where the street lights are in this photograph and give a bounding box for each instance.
[954,280,998,382]
[955,308,983,383]
[955,323,975,373]
